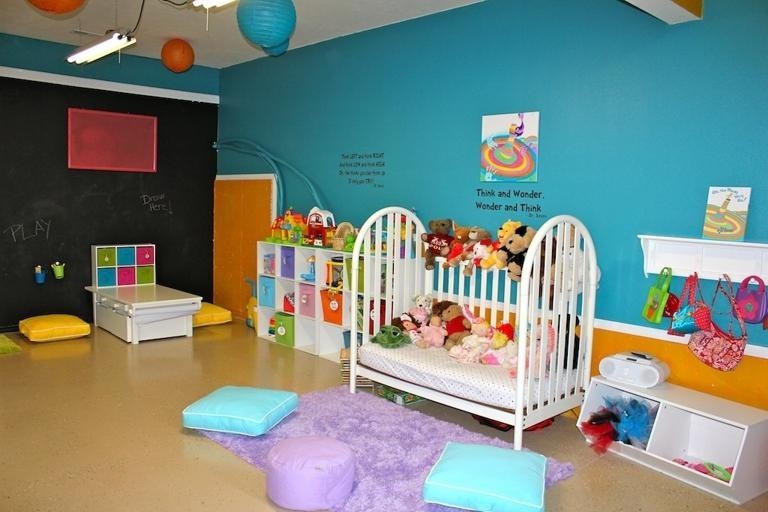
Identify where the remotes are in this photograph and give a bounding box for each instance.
[632,351,654,360]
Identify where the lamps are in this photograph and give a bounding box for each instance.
[64,0,137,65]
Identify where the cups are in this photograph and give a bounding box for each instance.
[35,271,45,284]
[53,265,65,279]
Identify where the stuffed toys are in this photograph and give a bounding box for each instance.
[375,292,552,381]
[419,217,576,293]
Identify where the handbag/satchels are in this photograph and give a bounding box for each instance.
[642,267,768,371]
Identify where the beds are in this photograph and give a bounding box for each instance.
[349,206,597,449]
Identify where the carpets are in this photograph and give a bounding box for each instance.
[199,381,575,512]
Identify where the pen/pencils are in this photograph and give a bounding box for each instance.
[50,262,66,268]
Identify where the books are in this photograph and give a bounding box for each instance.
[339,348,373,388]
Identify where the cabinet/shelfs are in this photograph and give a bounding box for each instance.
[256,240,428,364]
[576,373,768,506]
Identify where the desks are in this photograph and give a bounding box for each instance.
[84,284,204,345]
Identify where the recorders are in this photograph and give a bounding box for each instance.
[598,353,671,389]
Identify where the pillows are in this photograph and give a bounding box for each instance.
[181,385,299,438]
[192,301,232,327]
[422,440,548,511]
[18,314,91,343]
